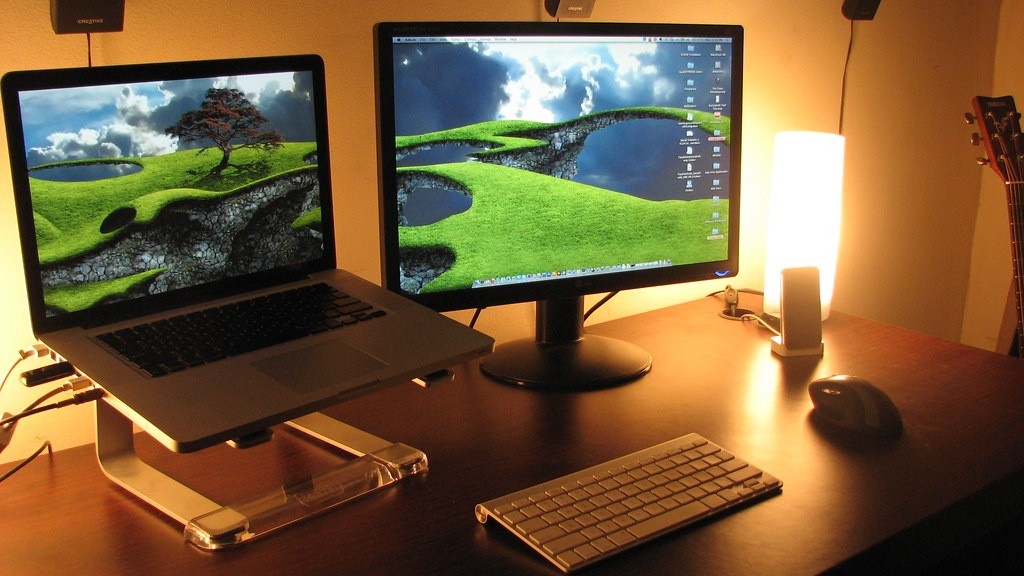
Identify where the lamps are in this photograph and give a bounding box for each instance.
[761,130,844,321]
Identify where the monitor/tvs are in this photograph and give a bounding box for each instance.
[372,21,741,393]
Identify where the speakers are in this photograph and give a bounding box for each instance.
[841,0,882,21]
[50,0,126,34]
[544,0,596,17]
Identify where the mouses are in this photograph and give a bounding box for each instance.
[808,373,905,437]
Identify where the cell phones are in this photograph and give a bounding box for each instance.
[779,266,822,351]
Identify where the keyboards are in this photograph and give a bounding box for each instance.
[474,433,786,574]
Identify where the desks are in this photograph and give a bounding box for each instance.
[0,289,1024,576]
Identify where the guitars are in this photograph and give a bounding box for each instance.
[959,93,1024,358]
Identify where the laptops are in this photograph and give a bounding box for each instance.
[1,53,495,456]
